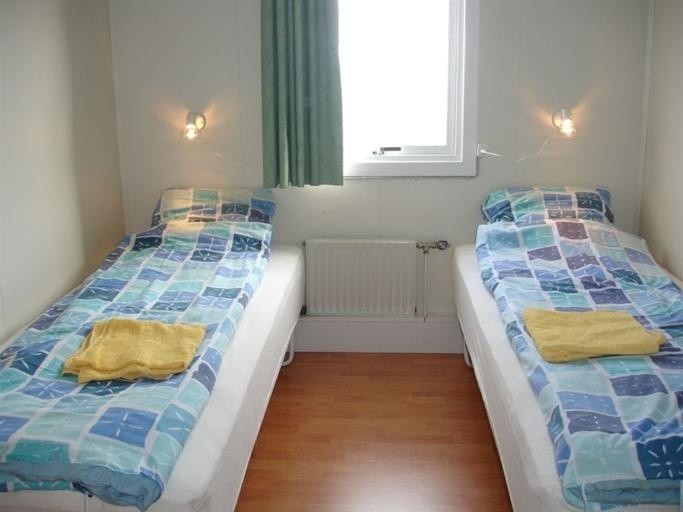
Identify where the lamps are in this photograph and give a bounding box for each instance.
[181,111,206,141]
[551,107,577,139]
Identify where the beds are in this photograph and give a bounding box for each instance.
[0,228,304,512]
[452,234,682,512]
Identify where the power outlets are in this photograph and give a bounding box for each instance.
[476,142,489,159]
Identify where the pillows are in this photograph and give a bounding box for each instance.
[150,186,278,226]
[480,186,613,233]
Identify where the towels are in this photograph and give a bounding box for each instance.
[519,306,668,364]
[58,316,206,385]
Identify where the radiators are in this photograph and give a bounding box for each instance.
[305,237,450,317]
